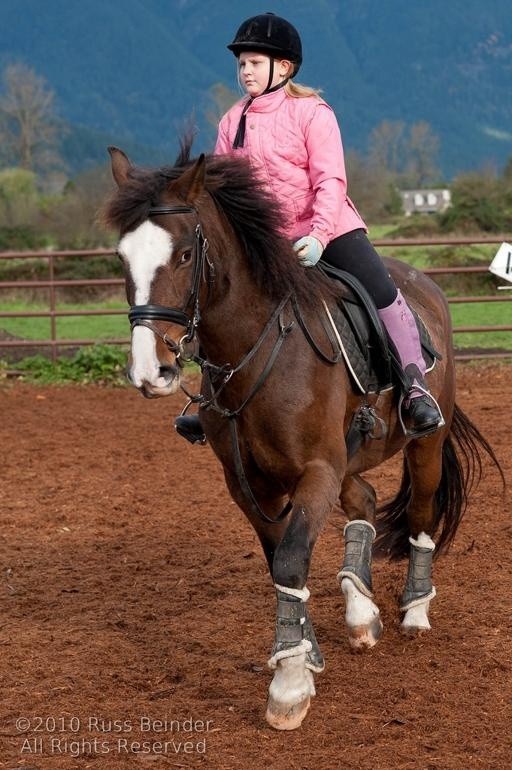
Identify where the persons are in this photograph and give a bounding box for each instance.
[172,11,442,435]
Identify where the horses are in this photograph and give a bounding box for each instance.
[89,106,507,732]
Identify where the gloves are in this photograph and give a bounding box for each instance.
[291,236,324,268]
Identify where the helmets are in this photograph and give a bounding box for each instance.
[227,12,302,66]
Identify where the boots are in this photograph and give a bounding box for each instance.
[377,288,440,431]
[173,414,207,446]
